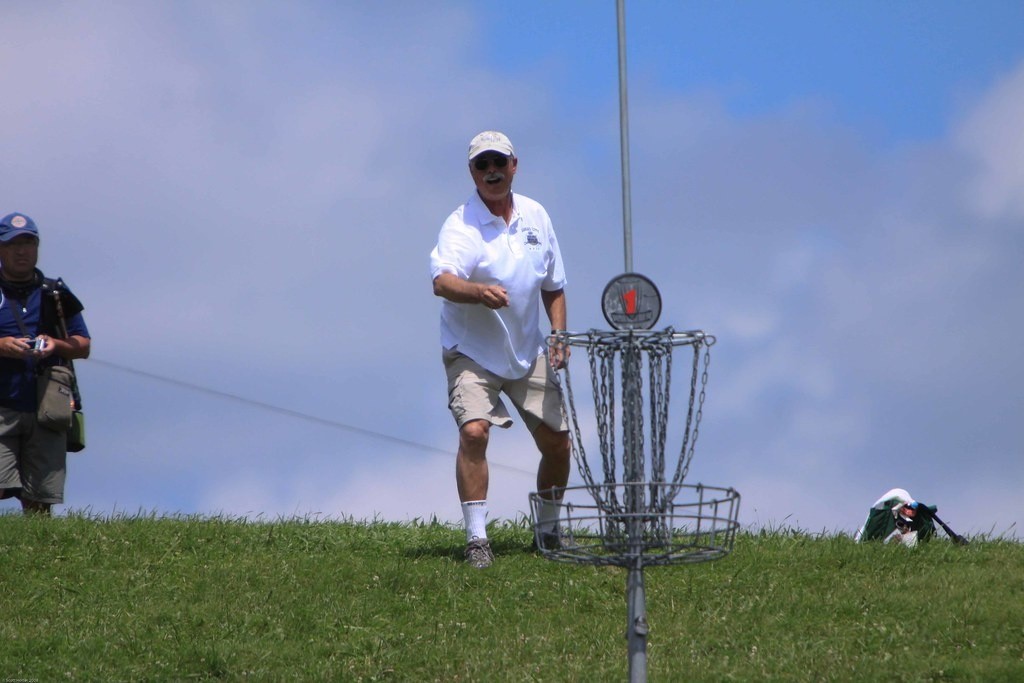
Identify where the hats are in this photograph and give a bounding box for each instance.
[0,212,39,243]
[468,131,515,161]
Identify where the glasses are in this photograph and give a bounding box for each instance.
[474,157,508,170]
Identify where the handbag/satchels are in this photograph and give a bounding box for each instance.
[913,502,937,542]
[50,277,86,453]
[6,293,78,435]
[853,488,915,548]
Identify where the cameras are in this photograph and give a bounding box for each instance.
[22,339,45,351]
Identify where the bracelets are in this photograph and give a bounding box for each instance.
[550,330,566,335]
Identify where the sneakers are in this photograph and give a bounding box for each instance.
[466,535,495,569]
[530,529,582,559]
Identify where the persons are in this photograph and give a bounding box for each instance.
[0,212,91,518]
[430,130,571,570]
